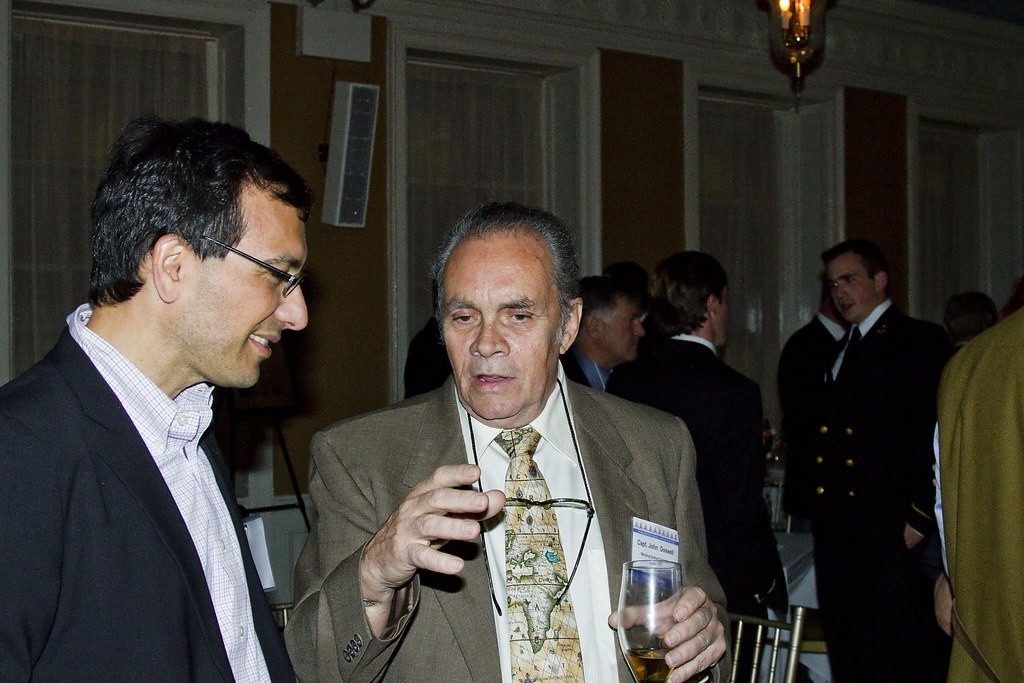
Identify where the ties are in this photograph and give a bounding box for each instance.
[494,426,590,683]
[834,326,862,381]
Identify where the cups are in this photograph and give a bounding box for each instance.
[617,560,684,683]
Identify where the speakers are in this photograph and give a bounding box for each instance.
[321,81,379,227]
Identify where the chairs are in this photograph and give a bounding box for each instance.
[729,607,806,683]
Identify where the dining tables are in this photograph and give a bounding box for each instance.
[761,531,817,680]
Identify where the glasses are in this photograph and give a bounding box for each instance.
[479,496,595,616]
[144,225,313,299]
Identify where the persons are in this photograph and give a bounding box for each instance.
[0,116,313,683]
[401,249,789,683]
[284,200,730,683]
[778,230,1024,683]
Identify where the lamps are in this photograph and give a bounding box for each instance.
[296,0,379,15]
[769,0,827,99]
